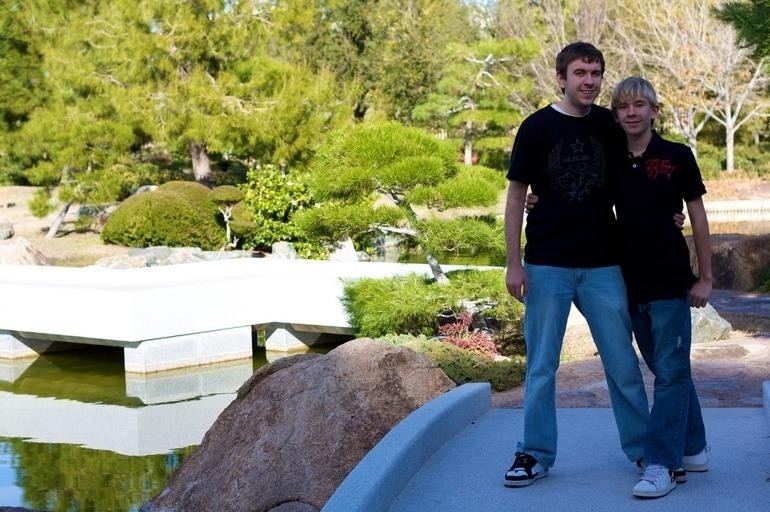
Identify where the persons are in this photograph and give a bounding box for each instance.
[526,77,712,498]
[502,42,687,487]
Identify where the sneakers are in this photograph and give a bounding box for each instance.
[633,460,687,500]
[503,454,549,487]
[683,445,710,472]
